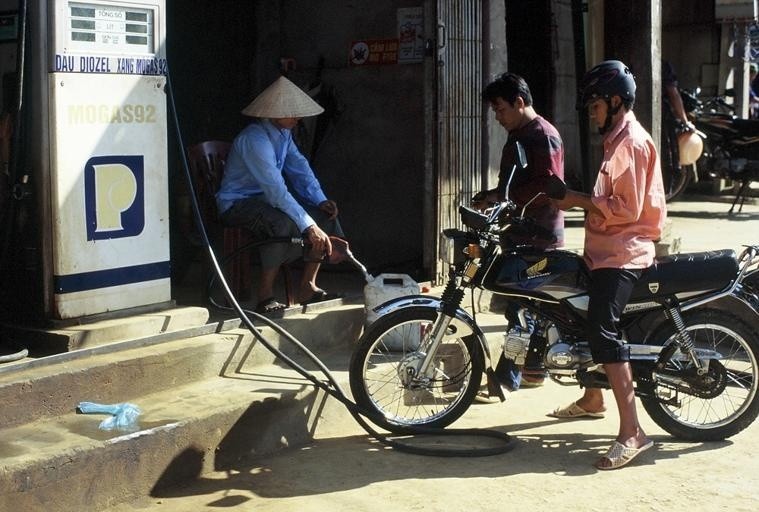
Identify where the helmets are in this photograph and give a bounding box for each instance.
[582,60,636,108]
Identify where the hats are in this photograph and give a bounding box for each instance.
[241,75,325,119]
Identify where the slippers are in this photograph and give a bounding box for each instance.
[257,297,287,312]
[301,288,344,306]
[551,401,603,418]
[475,382,511,403]
[520,376,544,386]
[597,439,654,470]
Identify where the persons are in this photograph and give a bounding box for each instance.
[750,62,758,118]
[662,55,695,202]
[469,75,566,404]
[543,59,669,468]
[212,74,344,314]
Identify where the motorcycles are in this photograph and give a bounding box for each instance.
[348,137,758,443]
[657,87,757,215]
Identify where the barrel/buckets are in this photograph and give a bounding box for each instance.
[364,273,421,352]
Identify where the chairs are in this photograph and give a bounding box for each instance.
[186,138,298,308]
